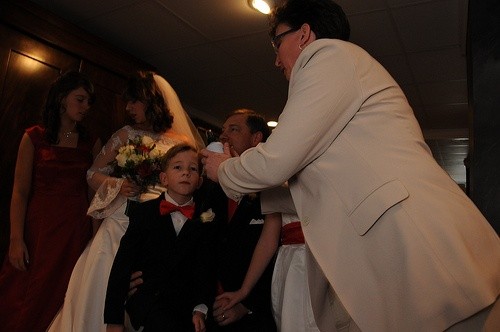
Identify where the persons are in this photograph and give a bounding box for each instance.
[128,109,279,332]
[201,0,500,332]
[0,73,101,332]
[216,179,320,332]
[47,70,206,332]
[104,143,223,332]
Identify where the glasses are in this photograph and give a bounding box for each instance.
[271,26,300,56]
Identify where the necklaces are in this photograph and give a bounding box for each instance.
[61,128,73,144]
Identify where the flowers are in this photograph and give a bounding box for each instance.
[111,135,166,191]
[191,208,215,223]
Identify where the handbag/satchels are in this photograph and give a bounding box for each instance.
[279,221,306,245]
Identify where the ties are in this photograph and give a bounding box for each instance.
[227,197,239,223]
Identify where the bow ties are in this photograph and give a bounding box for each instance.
[160,200,194,220]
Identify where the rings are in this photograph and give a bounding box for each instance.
[222,314,225,318]
